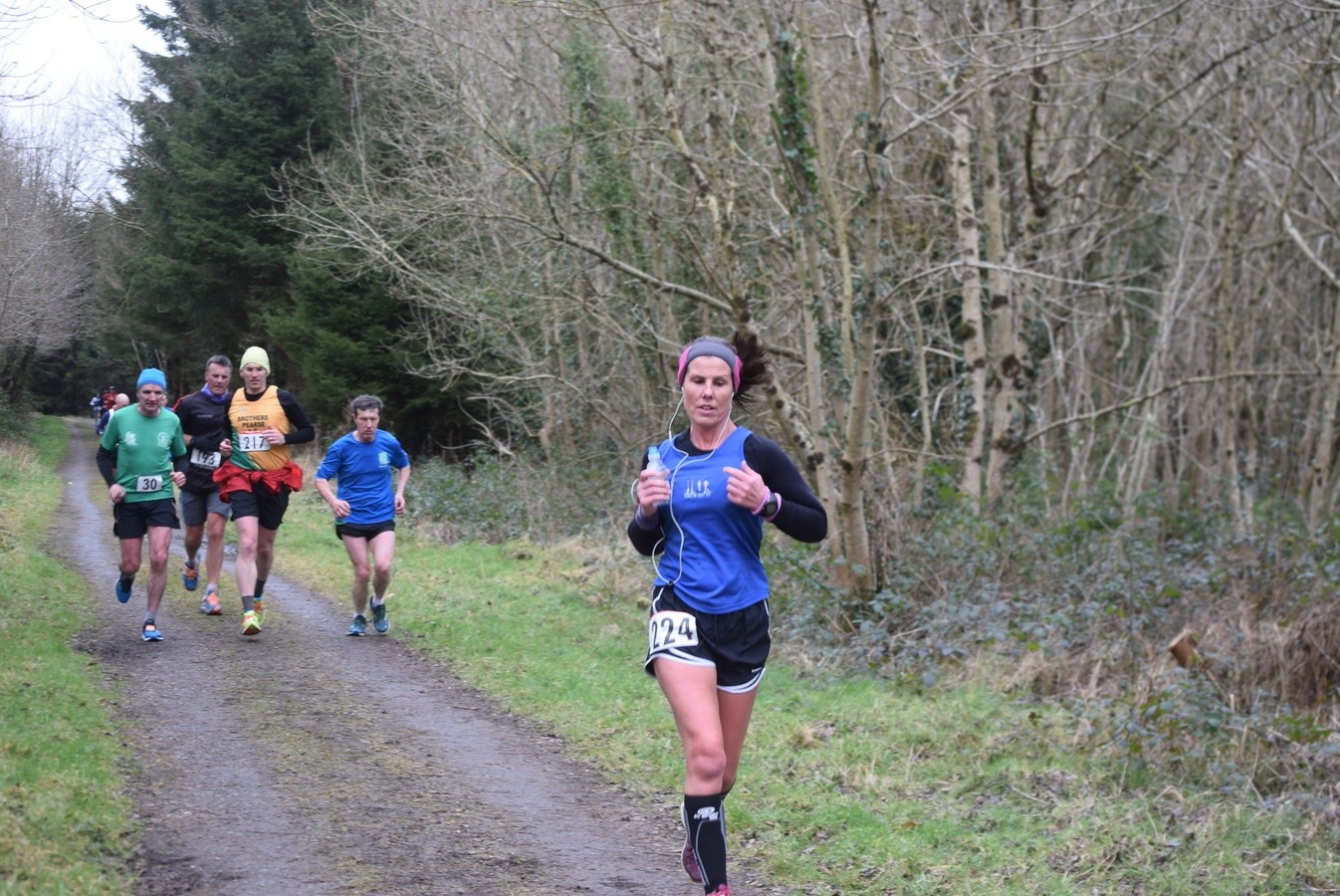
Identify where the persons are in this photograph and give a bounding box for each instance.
[171,353,234,614]
[96,393,130,480]
[626,330,828,896]
[212,345,315,636]
[90,386,116,427]
[313,394,411,636]
[95,368,189,641]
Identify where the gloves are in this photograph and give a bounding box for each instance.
[188,433,214,451]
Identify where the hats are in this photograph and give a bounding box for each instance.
[239,346,270,376]
[136,368,167,392]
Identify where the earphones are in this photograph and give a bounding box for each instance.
[731,389,737,398]
[680,386,685,395]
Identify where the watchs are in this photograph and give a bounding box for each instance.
[757,491,778,518]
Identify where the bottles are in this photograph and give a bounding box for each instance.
[644,446,672,508]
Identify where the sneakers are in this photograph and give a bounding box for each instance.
[254,591,265,625]
[200,595,223,615]
[370,595,390,633]
[346,615,368,637]
[183,555,200,590]
[116,571,135,603]
[242,610,262,635]
[680,801,705,882]
[141,619,164,642]
